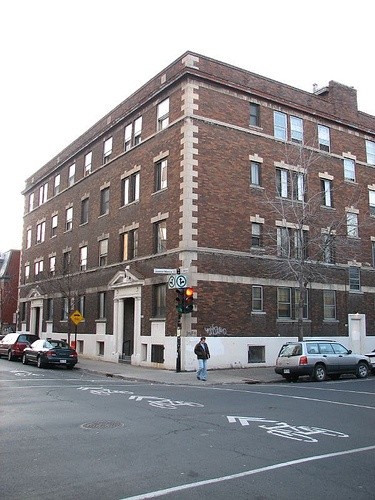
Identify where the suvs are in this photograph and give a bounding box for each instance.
[274,339,372,383]
[0,332,40,361]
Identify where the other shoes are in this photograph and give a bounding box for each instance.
[202,378,206,381]
[197,376,200,380]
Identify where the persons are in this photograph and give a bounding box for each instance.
[194,337,211,381]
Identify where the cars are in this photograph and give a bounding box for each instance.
[21,339,78,370]
[364,346,375,376]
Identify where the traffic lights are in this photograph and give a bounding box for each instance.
[175,288,184,313]
[184,287,193,313]
